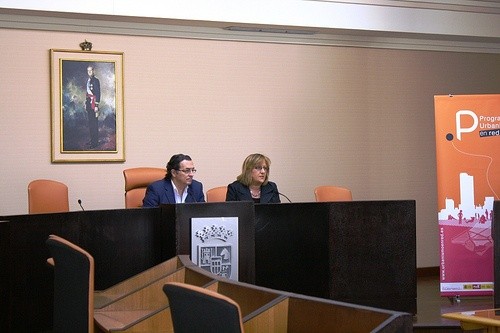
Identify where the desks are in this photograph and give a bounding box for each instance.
[439,308,500,333]
[0,200,418,333]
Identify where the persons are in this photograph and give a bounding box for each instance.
[143,153,206,207]
[225,154,281,204]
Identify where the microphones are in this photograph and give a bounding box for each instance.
[275,192,291,203]
[78,200,84,211]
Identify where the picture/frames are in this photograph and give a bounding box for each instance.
[49,48,126,164]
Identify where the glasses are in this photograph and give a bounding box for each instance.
[255,166,269,171]
[174,168,197,174]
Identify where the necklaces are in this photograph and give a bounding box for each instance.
[250,190,261,197]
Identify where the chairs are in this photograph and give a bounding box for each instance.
[163,281,245,333]
[28,168,353,214]
[47,234,94,333]
